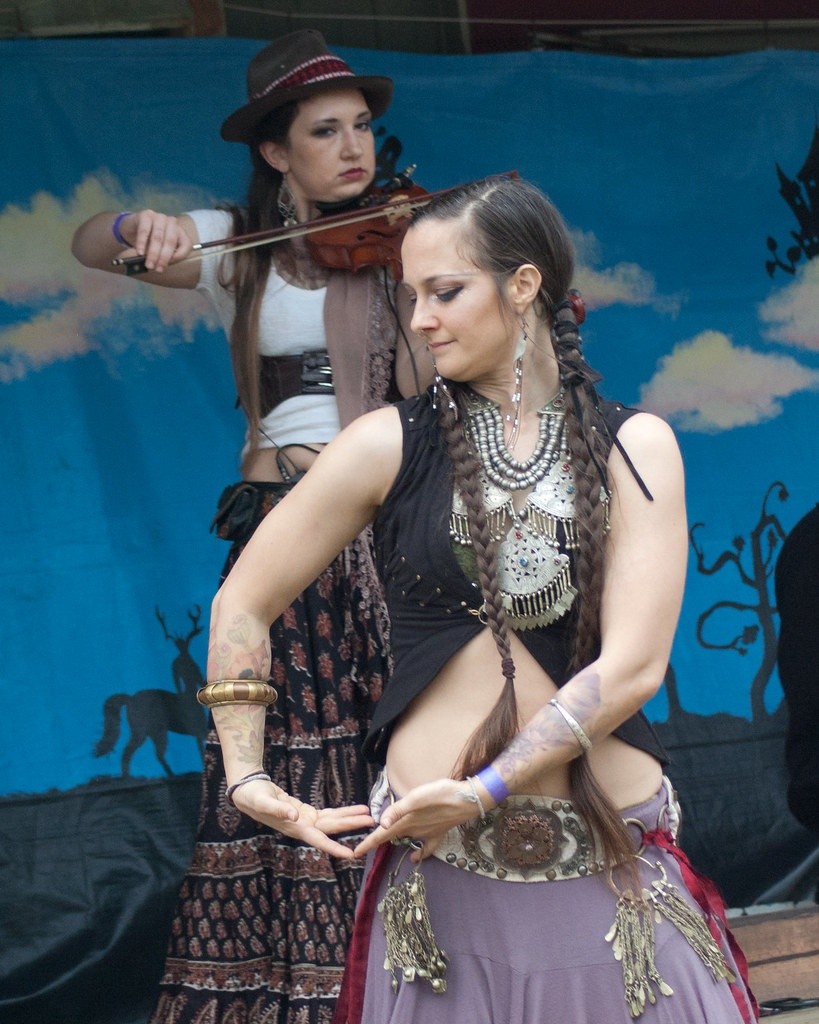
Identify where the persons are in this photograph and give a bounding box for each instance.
[71,28,438,1024]
[196,177,767,1024]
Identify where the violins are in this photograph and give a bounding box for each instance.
[304,184,587,326]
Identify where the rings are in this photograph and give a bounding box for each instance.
[400,836,412,845]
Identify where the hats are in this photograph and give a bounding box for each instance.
[219,29,395,142]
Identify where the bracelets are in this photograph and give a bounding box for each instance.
[112,212,136,250]
[476,763,510,804]
[458,776,486,820]
[548,699,594,754]
[197,679,279,707]
[224,770,272,806]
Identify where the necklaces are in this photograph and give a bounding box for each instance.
[443,377,613,633]
